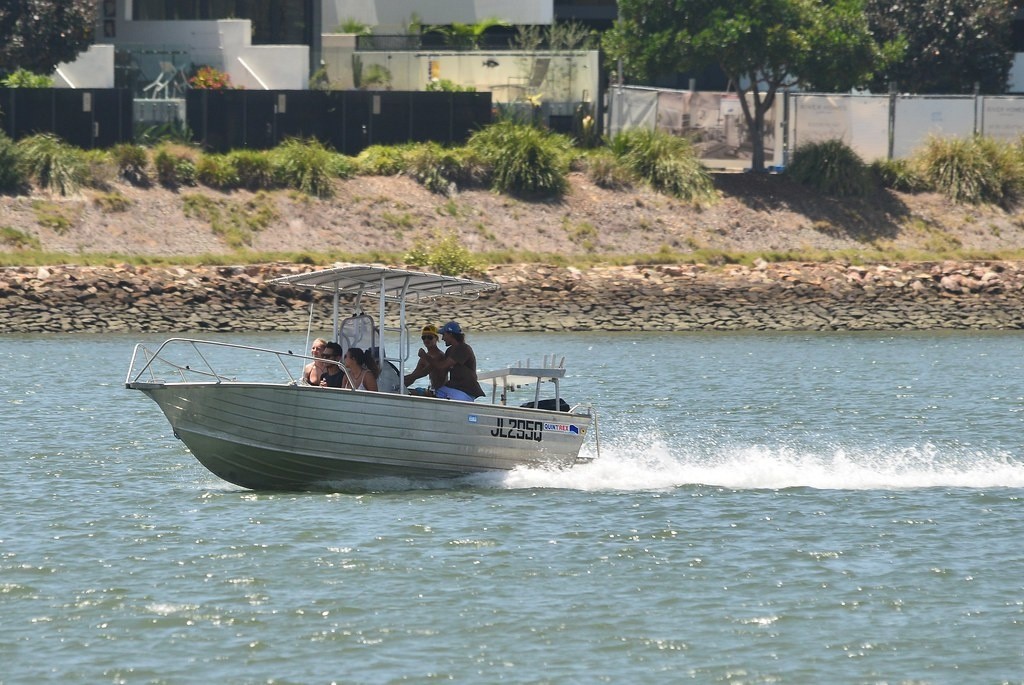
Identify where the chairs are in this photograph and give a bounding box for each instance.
[489,58,552,103]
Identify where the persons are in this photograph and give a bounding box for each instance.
[402,324,447,397]
[317,341,345,388]
[301,337,329,385]
[418,322,486,402]
[341,348,378,391]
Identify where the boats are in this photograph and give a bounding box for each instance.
[122,262,600,494]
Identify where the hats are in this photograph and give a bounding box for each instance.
[422,325,440,338]
[437,322,462,334]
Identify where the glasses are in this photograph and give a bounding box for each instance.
[422,335,433,340]
[344,354,354,358]
[321,353,338,358]
